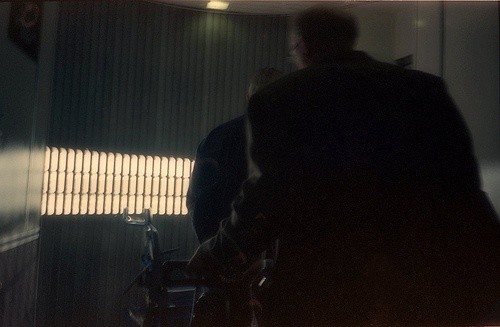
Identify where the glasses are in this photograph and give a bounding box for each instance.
[288,38,304,57]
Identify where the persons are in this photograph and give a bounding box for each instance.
[185,63,300,326]
[179,6,499,326]
